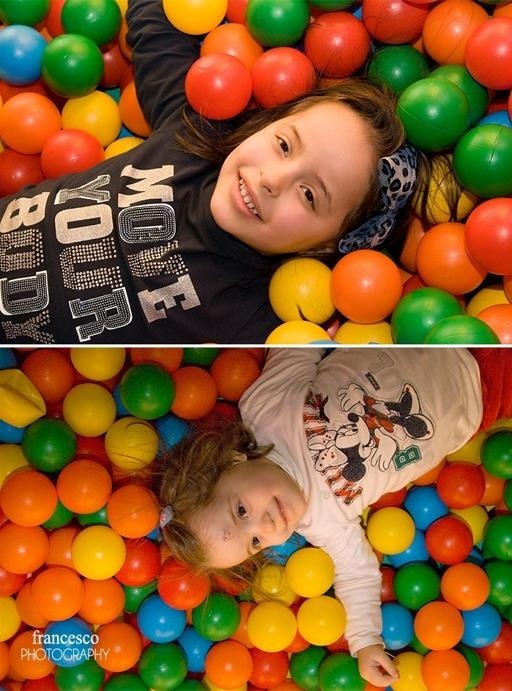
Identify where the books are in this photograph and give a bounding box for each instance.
[1,348,512,691]
[1,1,511,344]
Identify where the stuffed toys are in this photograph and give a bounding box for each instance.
[1,1,511,344]
[1,348,512,691]
